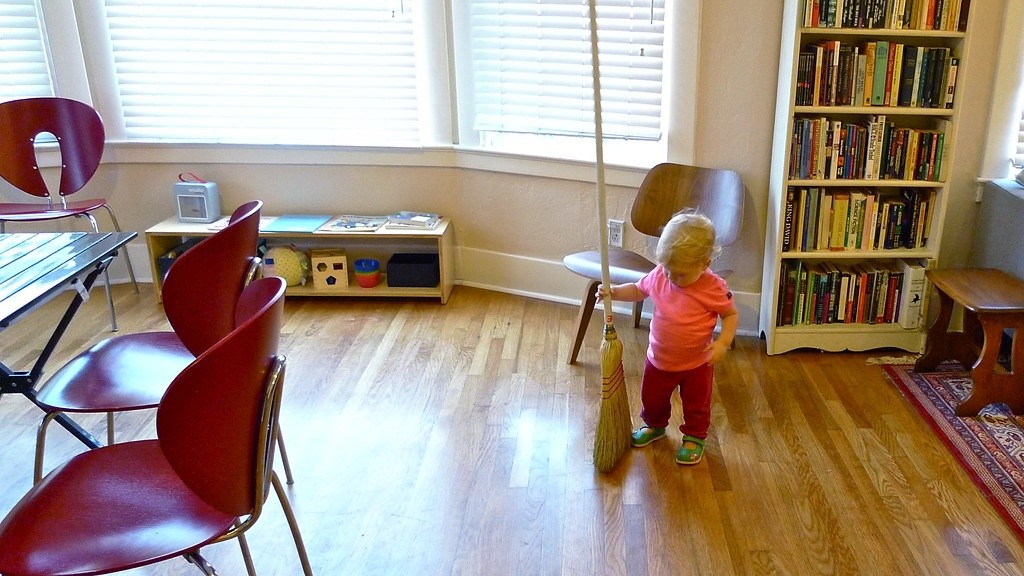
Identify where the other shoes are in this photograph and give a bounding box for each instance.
[676,436,704,464]
[631,422,668,448]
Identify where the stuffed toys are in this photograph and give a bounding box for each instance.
[261,242,311,287]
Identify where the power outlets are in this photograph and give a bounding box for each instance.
[608,218,626,248]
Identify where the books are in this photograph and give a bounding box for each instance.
[776,258,926,330]
[385,215,443,230]
[795,40,959,110]
[789,115,944,182]
[388,211,439,227]
[206,214,336,233]
[799,0,971,33]
[318,215,389,232]
[782,186,936,252]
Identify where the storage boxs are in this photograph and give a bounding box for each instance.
[311,247,349,289]
[386,253,440,288]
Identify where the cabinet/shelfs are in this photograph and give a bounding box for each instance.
[757,0,975,357]
[145,216,455,304]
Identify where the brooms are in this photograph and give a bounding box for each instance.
[587,0,632,470]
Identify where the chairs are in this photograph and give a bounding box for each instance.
[0,277,312,576]
[562,163,745,392]
[0,97,140,332]
[0,200,294,576]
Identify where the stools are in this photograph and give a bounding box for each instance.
[914,267,1024,416]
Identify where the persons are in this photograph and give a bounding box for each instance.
[595,206,739,465]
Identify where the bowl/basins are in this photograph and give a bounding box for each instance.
[354,258,380,288]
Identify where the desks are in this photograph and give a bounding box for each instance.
[0,232,216,576]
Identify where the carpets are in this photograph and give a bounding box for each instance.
[867,357,1024,545]
